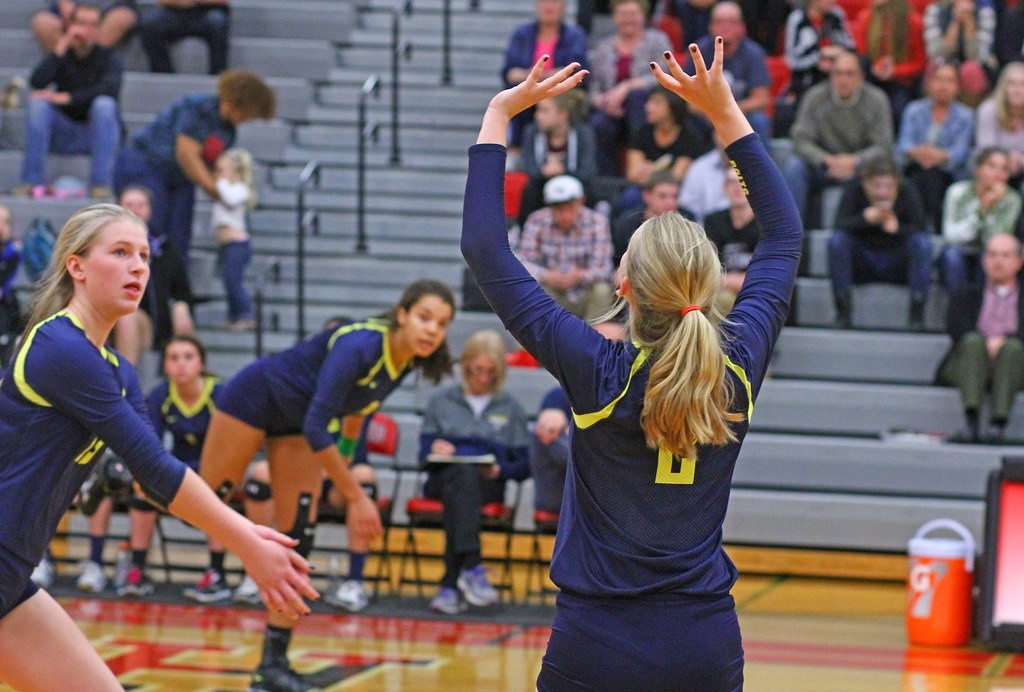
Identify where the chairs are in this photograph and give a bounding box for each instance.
[523,509,560,608]
[396,410,521,605]
[325,411,400,601]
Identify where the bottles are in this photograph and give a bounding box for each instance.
[112,543,129,589]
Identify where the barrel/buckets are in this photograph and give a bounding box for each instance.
[905,519,975,646]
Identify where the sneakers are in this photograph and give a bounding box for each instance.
[181,568,233,602]
[29,558,57,591]
[456,563,500,607]
[427,586,469,613]
[77,448,128,517]
[117,565,155,597]
[248,660,322,692]
[75,560,106,595]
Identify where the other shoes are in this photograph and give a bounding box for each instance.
[958,429,979,441]
[987,426,1000,441]
[326,580,367,611]
[8,183,33,196]
[232,573,263,605]
[90,185,112,199]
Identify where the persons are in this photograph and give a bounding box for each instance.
[76,279,457,692]
[500,0,1024,528]
[461,36,805,692]
[417,329,532,615]
[0,204,321,692]
[0,0,378,614]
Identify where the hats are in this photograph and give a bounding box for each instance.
[541,176,583,204]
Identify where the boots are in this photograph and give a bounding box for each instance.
[833,295,851,329]
[908,299,928,334]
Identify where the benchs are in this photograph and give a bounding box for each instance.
[0,0,1024,553]
